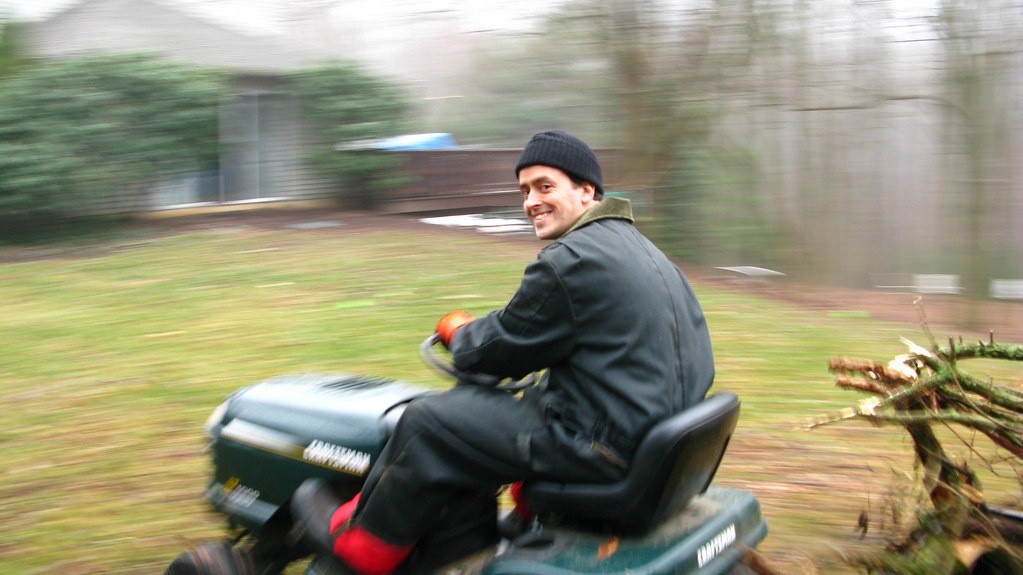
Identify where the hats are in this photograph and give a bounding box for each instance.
[515,129,604,199]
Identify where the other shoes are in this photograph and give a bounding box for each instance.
[295,479,344,549]
[497,510,527,542]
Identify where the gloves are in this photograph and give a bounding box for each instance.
[436,312,478,349]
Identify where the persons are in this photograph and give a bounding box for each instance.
[295,133,714,574]
